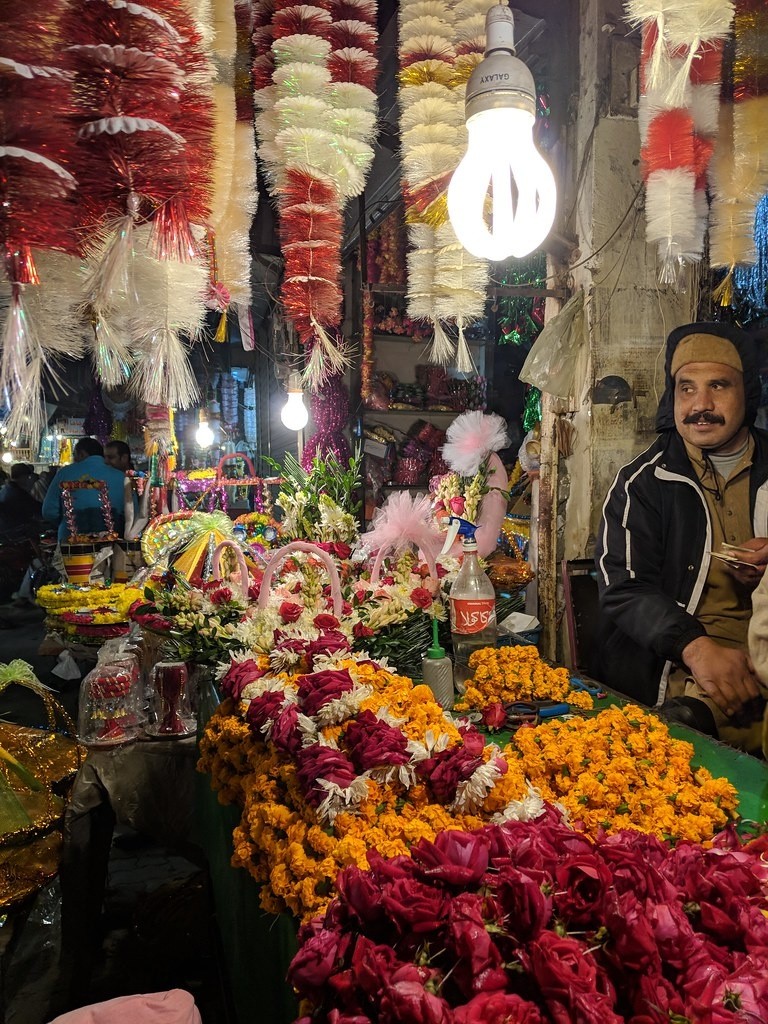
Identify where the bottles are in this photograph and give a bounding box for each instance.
[450,540,498,692]
[422,617,454,713]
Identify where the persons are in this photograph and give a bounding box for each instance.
[595,321,768,765]
[0,436,143,602]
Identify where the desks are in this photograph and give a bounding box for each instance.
[195,656,768,1024]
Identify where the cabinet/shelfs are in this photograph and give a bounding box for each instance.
[349,186,498,533]
